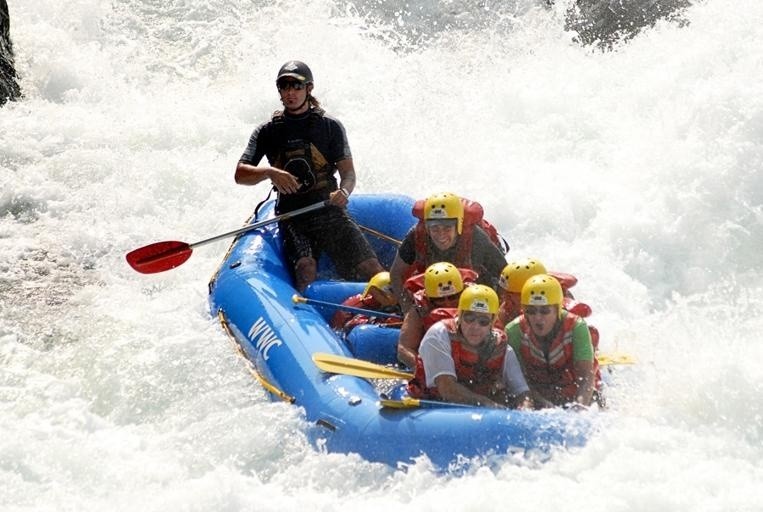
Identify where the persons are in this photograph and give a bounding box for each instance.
[398,261,479,370]
[390,192,508,315]
[405,284,535,410]
[332,271,405,331]
[503,274,595,412]
[235,61,386,295]
[497,257,599,350]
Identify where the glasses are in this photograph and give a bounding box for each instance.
[279,79,309,90]
[432,294,459,302]
[462,312,492,328]
[524,306,552,315]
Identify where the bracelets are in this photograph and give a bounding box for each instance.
[340,187,349,198]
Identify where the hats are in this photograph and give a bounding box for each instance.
[426,220,456,227]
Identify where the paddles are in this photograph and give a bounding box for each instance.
[594,353,634,367]
[126,199,331,273]
[312,353,415,380]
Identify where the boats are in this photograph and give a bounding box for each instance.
[206,190,606,479]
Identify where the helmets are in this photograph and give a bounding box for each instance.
[277,60,313,85]
[424,193,463,236]
[424,262,464,303]
[457,284,499,324]
[498,259,546,294]
[521,274,563,320]
[361,271,402,307]
